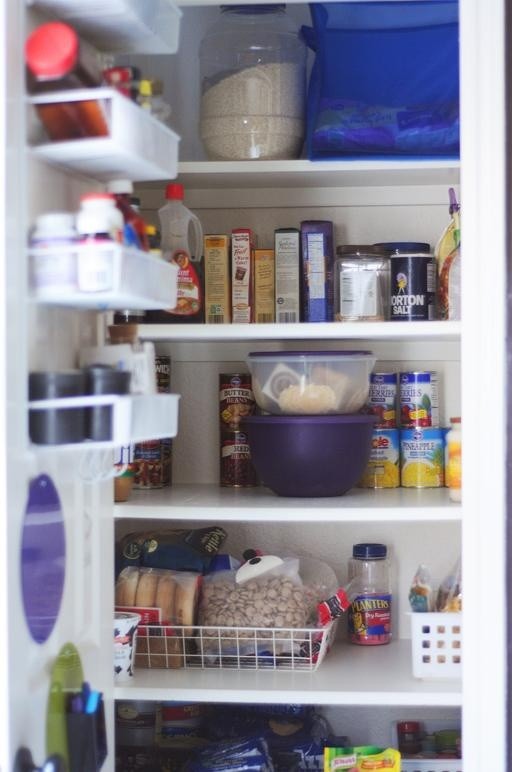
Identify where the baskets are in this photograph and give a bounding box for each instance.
[407,610,463,678]
[133,612,339,675]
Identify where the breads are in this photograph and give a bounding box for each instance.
[115,567,200,641]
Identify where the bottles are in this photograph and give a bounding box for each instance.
[198,3,309,162]
[444,416,464,505]
[23,20,116,143]
[29,207,78,298]
[76,192,125,294]
[25,367,134,445]
[346,541,393,649]
[106,178,150,256]
[142,182,210,324]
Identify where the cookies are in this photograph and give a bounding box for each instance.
[195,577,319,649]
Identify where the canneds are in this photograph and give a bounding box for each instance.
[131,355,174,490]
[219,372,254,431]
[331,242,436,321]
[397,722,460,759]
[220,430,256,488]
[361,371,452,488]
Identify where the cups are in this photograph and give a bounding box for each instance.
[113,609,143,683]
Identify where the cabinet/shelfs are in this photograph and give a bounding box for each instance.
[5,2,511,772]
